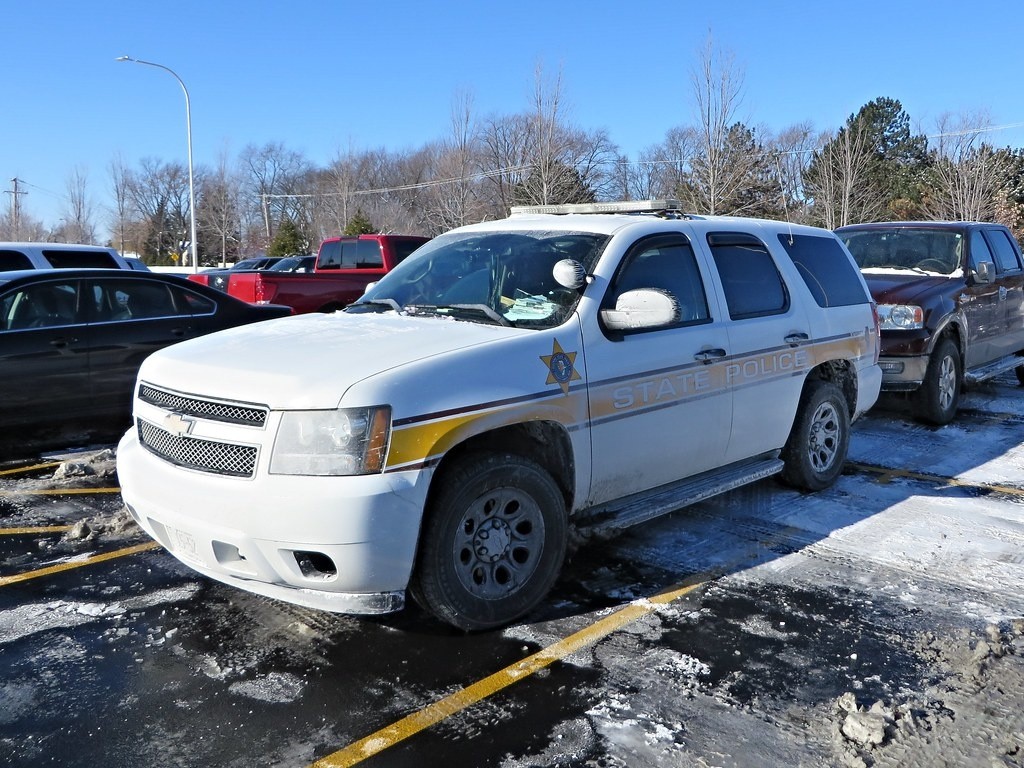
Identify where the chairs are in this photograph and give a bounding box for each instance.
[126,294,172,318]
[506,252,572,299]
[863,244,889,267]
[15,291,77,329]
[613,255,698,322]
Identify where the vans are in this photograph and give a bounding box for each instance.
[0,237,151,273]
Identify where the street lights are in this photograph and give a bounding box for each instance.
[113,54,200,266]
[59,217,93,246]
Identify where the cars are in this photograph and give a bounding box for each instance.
[0,268,298,469]
[197,255,318,297]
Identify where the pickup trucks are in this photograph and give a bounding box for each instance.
[186,233,433,318]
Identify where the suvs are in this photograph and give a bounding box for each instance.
[833,220,1022,426]
[114,199,884,633]
